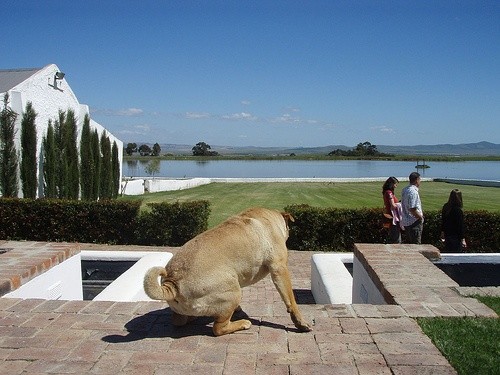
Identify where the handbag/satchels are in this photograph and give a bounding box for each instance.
[383,213,394,229]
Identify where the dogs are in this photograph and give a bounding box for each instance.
[144,208,314,337]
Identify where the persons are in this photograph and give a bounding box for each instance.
[440,188,467,253]
[382,176,401,243]
[402,172,424,244]
